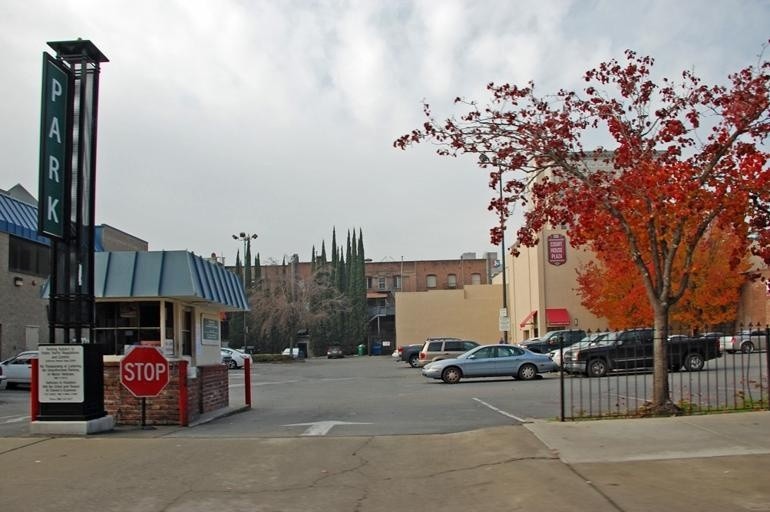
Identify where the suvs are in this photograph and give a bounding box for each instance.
[0,350,39,388]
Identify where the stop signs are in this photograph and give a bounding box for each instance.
[122,346,169,399]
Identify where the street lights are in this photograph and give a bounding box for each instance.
[493,153,513,346]
[232,233,259,353]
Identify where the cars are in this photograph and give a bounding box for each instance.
[220,345,257,369]
[327,345,343,359]
[283,347,304,360]
[0,363,8,391]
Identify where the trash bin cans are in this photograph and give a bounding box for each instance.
[358,345,365,357]
